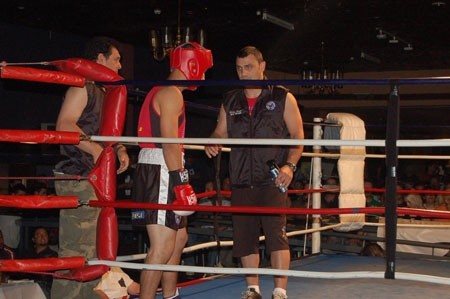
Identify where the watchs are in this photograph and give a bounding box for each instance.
[285,161,296,171]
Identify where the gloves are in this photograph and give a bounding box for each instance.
[168,168,197,216]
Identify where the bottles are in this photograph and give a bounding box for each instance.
[266,159,288,192]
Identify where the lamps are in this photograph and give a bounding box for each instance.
[360,52,381,64]
[296,38,344,97]
[150,0,204,63]
[377,30,413,50]
[262,13,294,31]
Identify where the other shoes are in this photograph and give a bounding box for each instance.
[243,288,262,299]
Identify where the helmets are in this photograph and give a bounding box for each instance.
[170,42,213,91]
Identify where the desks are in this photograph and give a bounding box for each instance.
[0,282,46,299]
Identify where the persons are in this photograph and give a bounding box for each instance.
[205,47,304,299]
[397,185,450,222]
[287,177,340,208]
[23,225,58,259]
[364,180,382,208]
[92,266,140,299]
[50,38,129,299]
[130,43,213,299]
[198,177,232,207]
[0,230,15,260]
[0,183,49,217]
